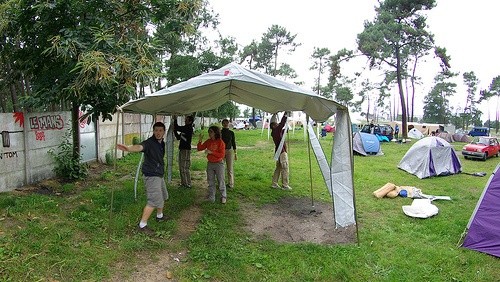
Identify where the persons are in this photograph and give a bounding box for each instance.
[369,120,401,141]
[219,118,239,189]
[270,109,294,190]
[174,112,196,188]
[197,124,228,205]
[116,122,171,237]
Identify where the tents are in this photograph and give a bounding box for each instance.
[353,130,385,158]
[437,131,454,142]
[108,61,363,248]
[407,127,424,140]
[457,162,500,260]
[397,137,463,179]
[454,133,470,142]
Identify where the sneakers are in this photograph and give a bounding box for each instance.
[179,183,293,203]
[135,224,155,236]
[156,214,172,221]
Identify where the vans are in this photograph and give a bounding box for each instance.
[468,128,489,136]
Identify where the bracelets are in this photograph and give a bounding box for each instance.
[235,152,238,154]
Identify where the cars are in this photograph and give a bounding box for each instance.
[361,124,394,141]
[462,137,500,161]
